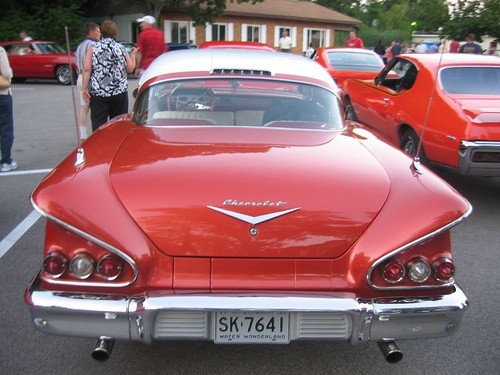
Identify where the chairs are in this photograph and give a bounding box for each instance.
[150,110,235,125]
[260,98,331,129]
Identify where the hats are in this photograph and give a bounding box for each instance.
[136,15,157,24]
[464,33,475,39]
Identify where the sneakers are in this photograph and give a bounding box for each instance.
[1,161,17,171]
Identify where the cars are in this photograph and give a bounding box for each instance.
[2,42,78,86]
[344,53,500,184]
[28,48,472,362]
[303,49,385,84]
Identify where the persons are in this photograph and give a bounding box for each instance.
[0,46,18,172]
[136,16,167,80]
[306,43,316,61]
[373,34,500,64]
[82,20,139,132]
[279,31,293,53]
[75,22,101,140]
[346,29,363,48]
[20,30,33,41]
